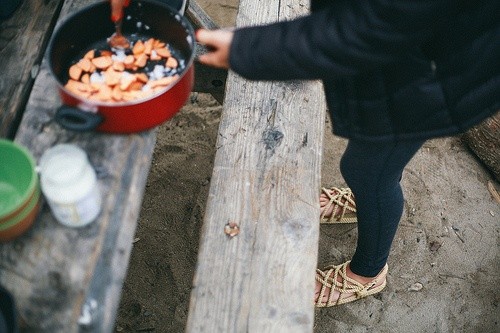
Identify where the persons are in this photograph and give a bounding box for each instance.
[110,0,500,307]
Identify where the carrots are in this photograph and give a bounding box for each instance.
[65,37,179,104]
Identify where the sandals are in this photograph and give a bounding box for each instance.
[320,186,358,224]
[314,260,389,307]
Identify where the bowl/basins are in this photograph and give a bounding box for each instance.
[0,139,40,242]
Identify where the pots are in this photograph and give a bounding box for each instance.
[45,0,196,134]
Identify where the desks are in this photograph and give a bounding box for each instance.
[0,0,228,333]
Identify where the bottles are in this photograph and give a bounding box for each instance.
[37,145,103,229]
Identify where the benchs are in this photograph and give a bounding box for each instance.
[186,0,324,333]
